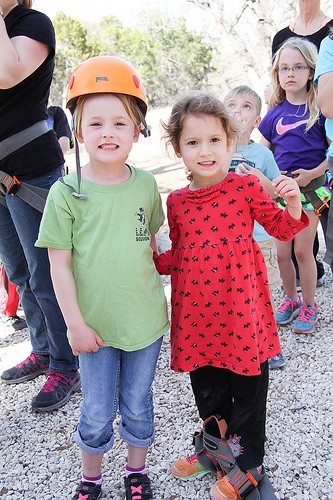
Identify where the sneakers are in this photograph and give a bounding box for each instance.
[290,301,320,334]
[122,473,153,500]
[71,480,102,500]
[274,293,302,325]
[171,448,217,482]
[209,464,267,500]
[0,352,50,385]
[31,370,83,414]
[268,352,286,370]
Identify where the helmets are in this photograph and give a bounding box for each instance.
[65,55,150,118]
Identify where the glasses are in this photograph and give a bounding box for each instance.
[279,65,309,73]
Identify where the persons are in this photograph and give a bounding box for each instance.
[0,105,74,330]
[0,0,82,412]
[151,91,311,500]
[34,55,166,500]
[222,0,333,370]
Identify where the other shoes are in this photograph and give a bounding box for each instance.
[296,275,324,292]
[0,313,28,332]
